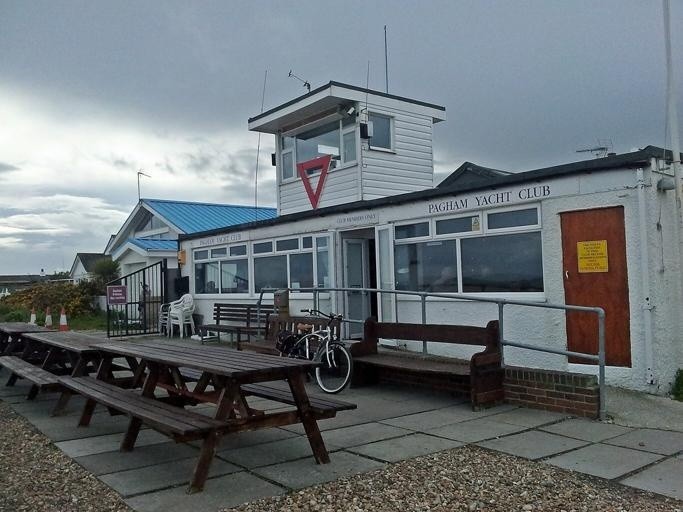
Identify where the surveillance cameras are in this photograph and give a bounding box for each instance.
[347,107,355,116]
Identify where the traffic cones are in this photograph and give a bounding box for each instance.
[58,307,69,332]
[41,306,52,330]
[27,306,38,326]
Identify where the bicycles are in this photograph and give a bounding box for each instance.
[278,308,353,395]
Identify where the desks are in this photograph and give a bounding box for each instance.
[0,321,57,371]
[6,330,137,417]
[76,338,330,495]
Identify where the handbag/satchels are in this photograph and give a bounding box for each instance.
[275,330,294,354]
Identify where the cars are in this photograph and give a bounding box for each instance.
[0,286,12,302]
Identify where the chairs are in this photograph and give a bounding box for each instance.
[159,294,196,338]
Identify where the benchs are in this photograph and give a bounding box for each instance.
[56,375,227,442]
[236,314,342,378]
[1,354,60,389]
[347,315,506,412]
[198,302,280,351]
[114,358,357,416]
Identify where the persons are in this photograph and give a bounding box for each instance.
[427,267,457,292]
